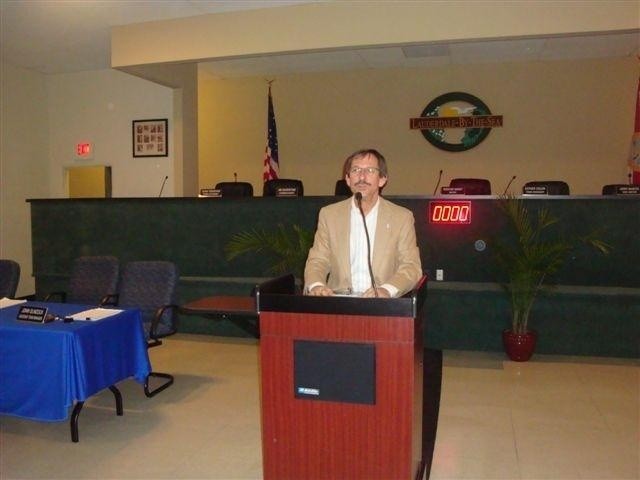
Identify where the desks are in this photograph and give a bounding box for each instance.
[24,195,639,360]
[0,299,152,442]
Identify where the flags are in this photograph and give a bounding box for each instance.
[264,86,280,185]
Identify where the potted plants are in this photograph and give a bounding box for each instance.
[485,189,615,362]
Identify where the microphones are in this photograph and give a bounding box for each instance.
[433,170,442,196]
[234,172,238,182]
[502,176,515,196]
[355,191,380,298]
[628,173,632,193]
[159,176,168,197]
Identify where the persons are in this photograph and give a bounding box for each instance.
[300,147,422,308]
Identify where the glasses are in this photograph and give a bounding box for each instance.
[348,166,380,175]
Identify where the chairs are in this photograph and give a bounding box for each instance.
[335,180,353,195]
[99,260,178,398]
[43,256,119,307]
[449,178,491,195]
[602,184,640,196]
[523,181,570,195]
[215,182,253,197]
[263,179,303,197]
[0,259,20,300]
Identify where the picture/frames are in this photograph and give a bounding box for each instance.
[132,118,168,158]
